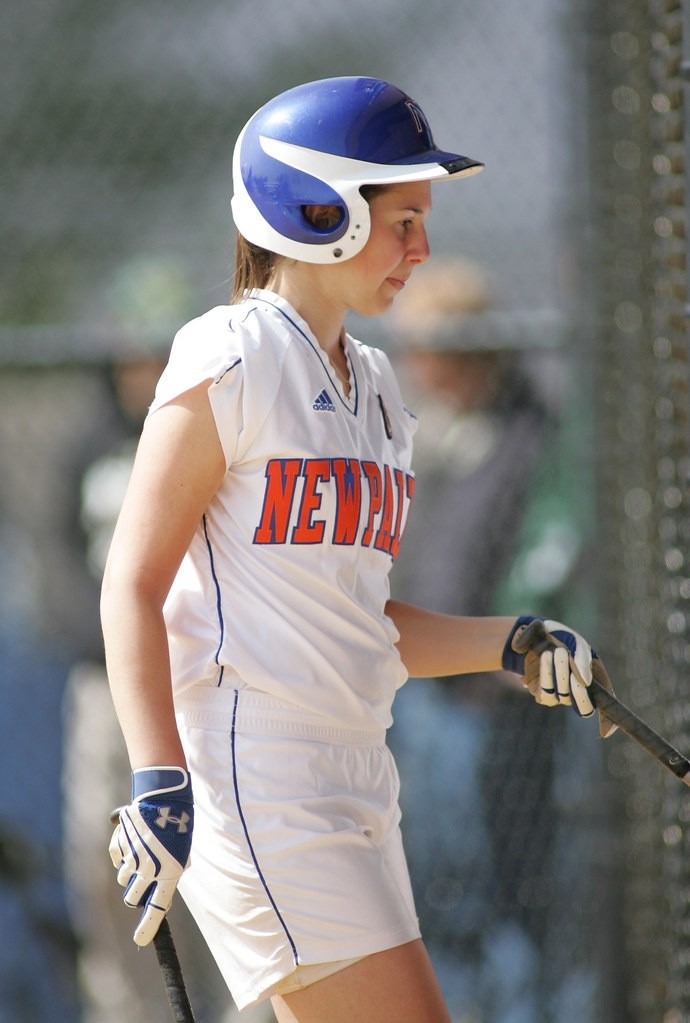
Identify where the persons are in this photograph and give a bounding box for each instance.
[31,72,565,1022]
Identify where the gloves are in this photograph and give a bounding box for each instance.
[503,616,622,739]
[109,764,194,947]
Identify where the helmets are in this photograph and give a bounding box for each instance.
[230,75,485,267]
